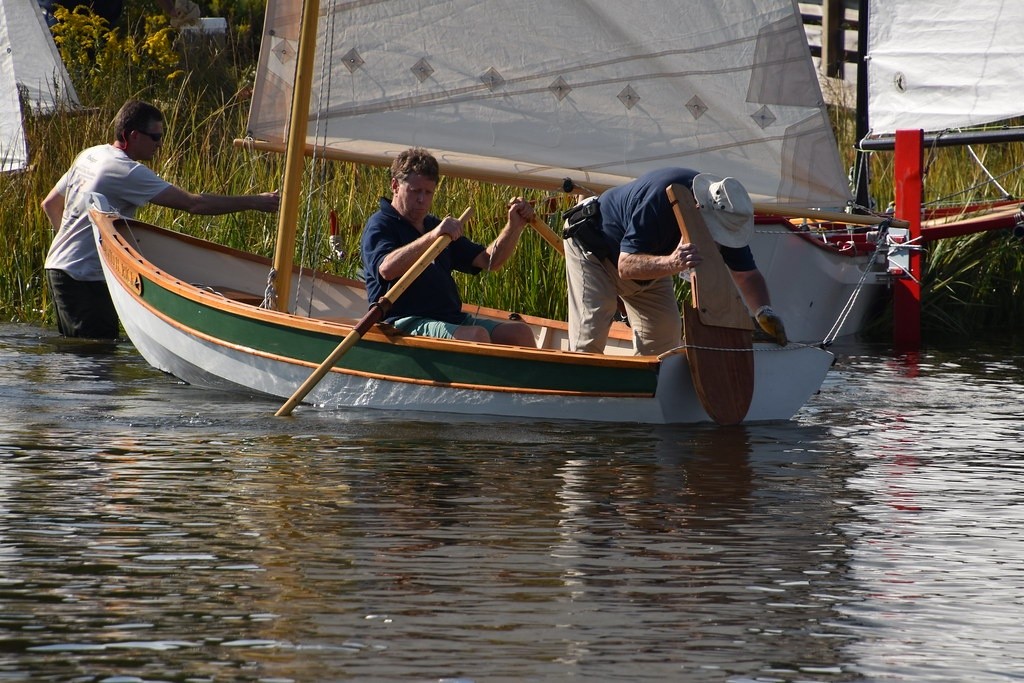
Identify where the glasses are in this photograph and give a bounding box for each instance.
[138,130,162,142]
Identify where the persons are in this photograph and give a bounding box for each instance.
[42,100,279,340]
[562,168,787,355]
[362,149,536,348]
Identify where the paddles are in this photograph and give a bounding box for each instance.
[274,204,477,420]
[508,194,562,253]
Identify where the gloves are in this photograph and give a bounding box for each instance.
[755,305,787,347]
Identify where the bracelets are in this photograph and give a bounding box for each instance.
[755,306,773,323]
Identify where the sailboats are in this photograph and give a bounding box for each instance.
[82,1,1023,429]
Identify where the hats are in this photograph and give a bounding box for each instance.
[693,173,754,248]
